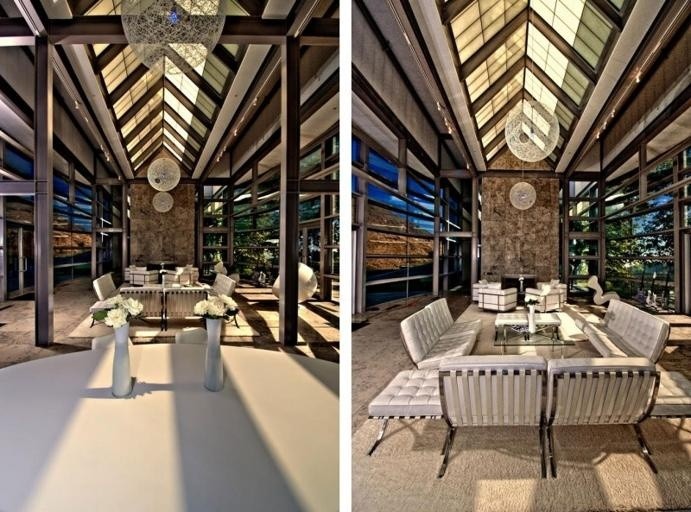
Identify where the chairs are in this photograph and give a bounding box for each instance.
[90,265,239,350]
[472,279,568,313]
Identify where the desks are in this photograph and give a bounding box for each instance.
[0,343,339,511]
